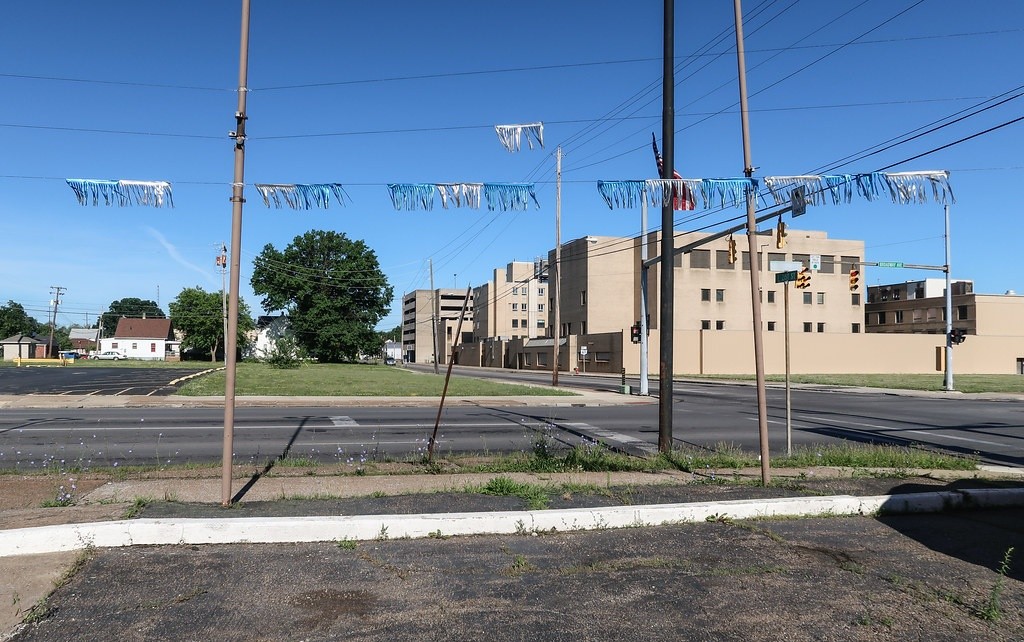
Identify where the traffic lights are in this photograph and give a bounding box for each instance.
[850,269,858,291]
[631,325,639,344]
[777,222,789,248]
[728,240,737,264]
[802,267,811,287]
[958,329,967,344]
[794,273,804,288]
[950,328,957,342]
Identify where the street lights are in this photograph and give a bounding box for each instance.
[453,273,457,289]
[428,259,434,290]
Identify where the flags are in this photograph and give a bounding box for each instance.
[653,140,695,211]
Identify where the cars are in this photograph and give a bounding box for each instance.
[90,351,127,361]
[78,355,90,360]
[74,353,79,359]
[384,357,396,366]
